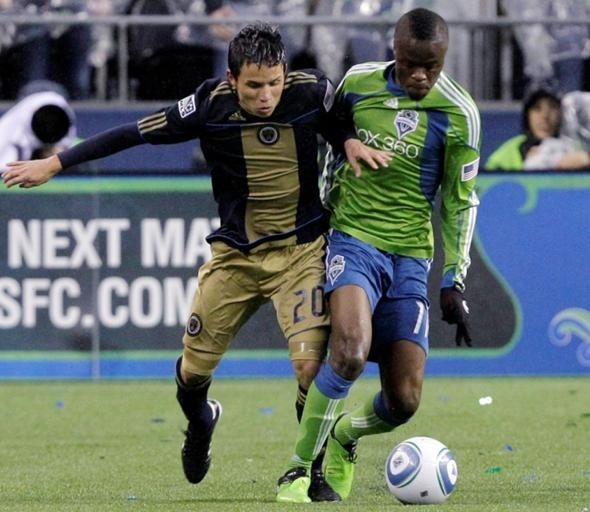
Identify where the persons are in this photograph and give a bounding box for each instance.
[1,1,589,177]
[274,9,483,504]
[0,22,396,484]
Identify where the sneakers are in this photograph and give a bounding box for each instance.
[275,464,312,504]
[181,399,222,484]
[308,464,342,504]
[322,410,358,500]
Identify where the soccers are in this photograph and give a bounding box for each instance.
[384,438,458,504]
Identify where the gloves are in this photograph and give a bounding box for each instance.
[439,288,474,348]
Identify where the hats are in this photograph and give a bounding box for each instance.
[522,76,570,108]
